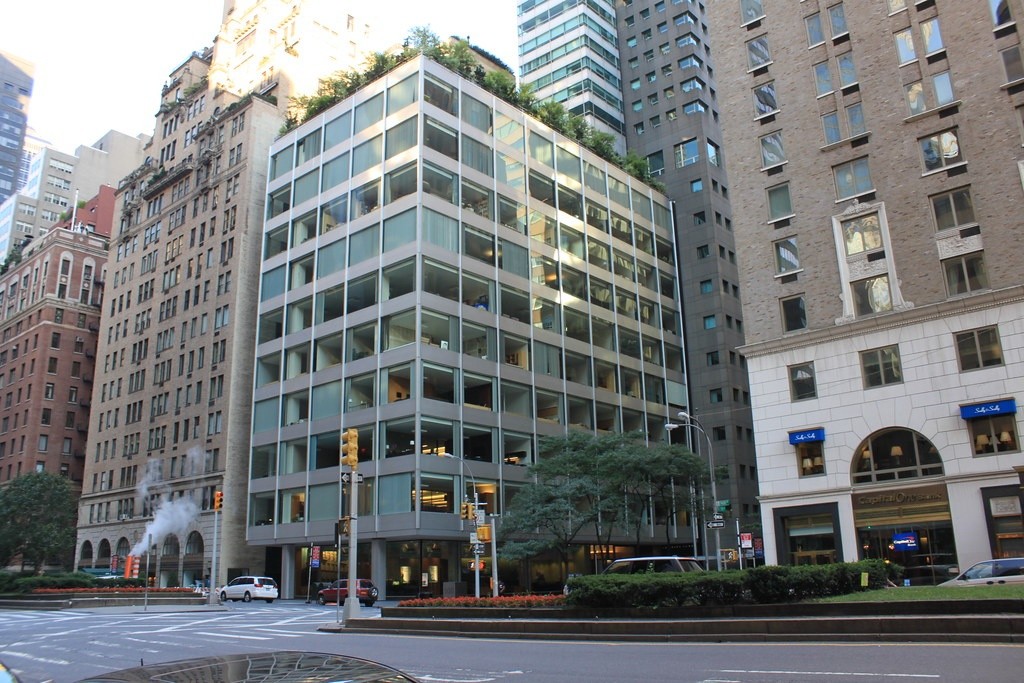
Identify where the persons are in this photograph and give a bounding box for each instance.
[498,579,505,596]
[885,567,898,588]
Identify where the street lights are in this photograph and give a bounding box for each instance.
[664,411,724,574]
[443,452,480,599]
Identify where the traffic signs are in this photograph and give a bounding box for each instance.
[706,521,725,529]
[340,471,363,484]
[472,543,485,550]
[472,550,485,555]
[713,513,724,520]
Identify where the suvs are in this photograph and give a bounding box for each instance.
[219,576,279,604]
[563,556,706,597]
[318,578,378,607]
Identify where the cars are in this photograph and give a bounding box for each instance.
[937,557,1024,587]
[185,584,219,597]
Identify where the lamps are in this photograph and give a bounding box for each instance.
[862,451,872,472]
[802,457,824,475]
[974,432,1013,454]
[891,446,903,468]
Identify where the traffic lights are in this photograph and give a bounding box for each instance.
[338,518,350,535]
[724,549,738,562]
[214,490,223,510]
[461,503,469,521]
[468,560,475,571]
[478,559,486,571]
[341,428,357,467]
[468,503,475,520]
